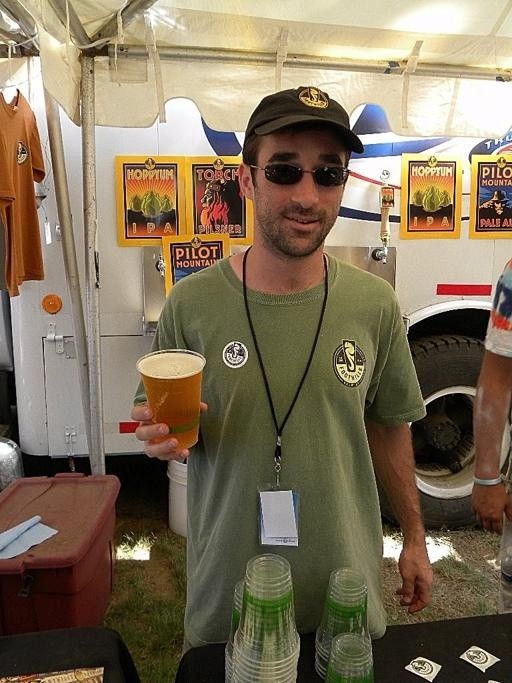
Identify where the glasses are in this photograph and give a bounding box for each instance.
[247,162,351,187]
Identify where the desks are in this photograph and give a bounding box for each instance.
[1,626,142,683]
[176,613,511,682]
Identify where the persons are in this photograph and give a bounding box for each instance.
[468,252,512,610]
[124,83,435,661]
[198,178,242,234]
[479,188,511,226]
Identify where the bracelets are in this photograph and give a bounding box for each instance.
[472,473,504,487]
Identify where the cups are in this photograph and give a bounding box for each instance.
[223,551,376,683]
[135,348,207,452]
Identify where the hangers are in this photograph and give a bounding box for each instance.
[0,43,21,96]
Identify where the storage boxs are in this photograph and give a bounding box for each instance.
[1,472,123,637]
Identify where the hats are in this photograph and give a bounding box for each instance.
[243,86,366,155]
